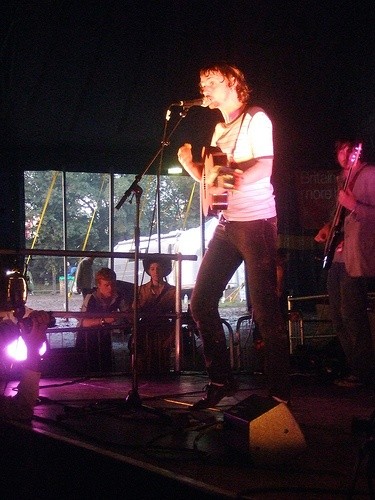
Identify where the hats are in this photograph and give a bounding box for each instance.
[143,253,172,277]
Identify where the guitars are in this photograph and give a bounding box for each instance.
[200,144,242,216]
[322,143,363,271]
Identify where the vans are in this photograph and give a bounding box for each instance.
[107,217,238,291]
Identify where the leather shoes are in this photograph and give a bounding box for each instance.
[188,380,240,412]
[269,395,289,406]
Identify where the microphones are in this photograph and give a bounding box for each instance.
[175,97,211,107]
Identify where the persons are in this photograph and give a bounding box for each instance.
[314,136,375,389]
[176,59,295,410]
[0,311,50,419]
[129,254,184,371]
[77,267,133,345]
[77,250,97,298]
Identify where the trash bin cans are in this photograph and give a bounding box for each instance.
[59,276,75,296]
